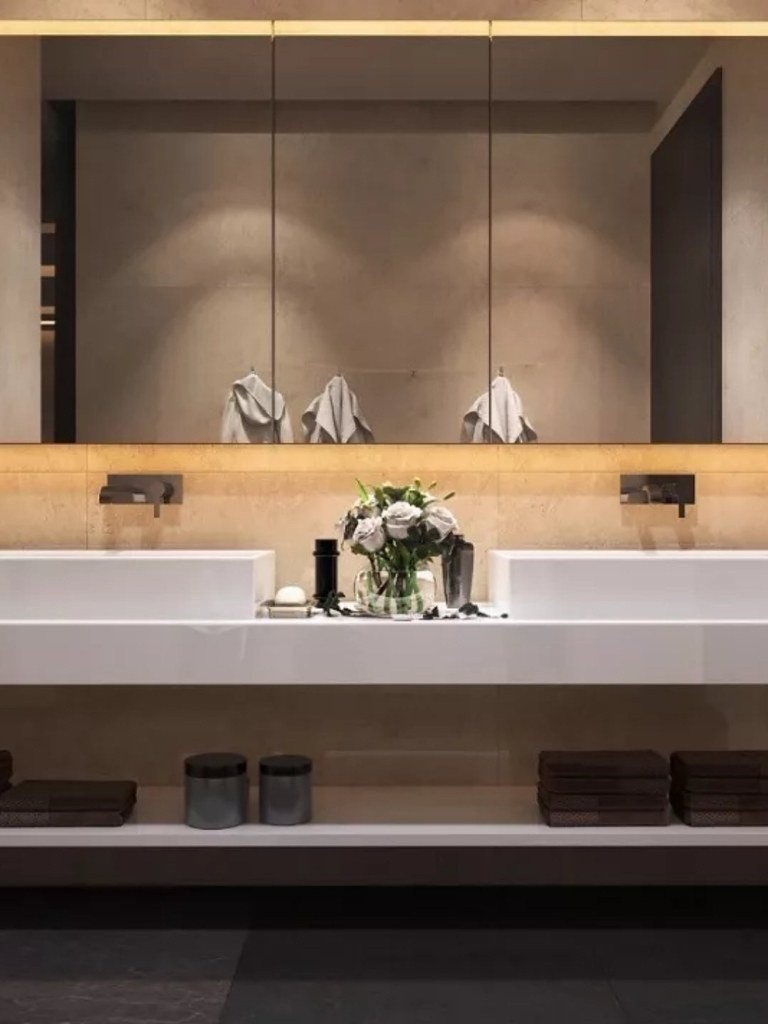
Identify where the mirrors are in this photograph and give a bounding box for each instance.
[0,32,768,445]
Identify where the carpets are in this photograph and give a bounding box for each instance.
[218,887,767,1023]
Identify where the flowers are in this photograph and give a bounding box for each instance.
[335,474,460,570]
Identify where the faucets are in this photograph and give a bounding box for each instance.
[151,480,171,518]
[671,481,687,520]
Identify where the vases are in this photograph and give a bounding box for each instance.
[353,568,437,620]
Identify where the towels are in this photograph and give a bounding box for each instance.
[463,376,539,445]
[219,371,295,443]
[301,375,376,443]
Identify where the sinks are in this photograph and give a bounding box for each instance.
[2,544,268,621]
[487,549,765,620]
